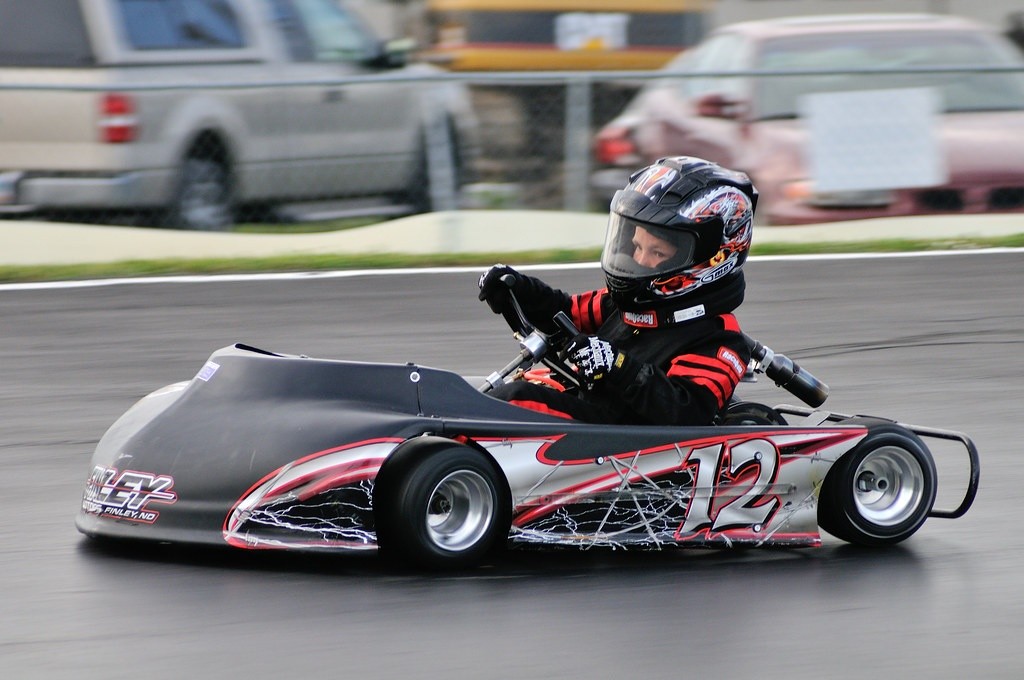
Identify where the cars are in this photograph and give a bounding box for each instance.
[591,15,1024,229]
[0,1,484,235]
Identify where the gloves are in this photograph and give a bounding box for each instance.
[478,262,532,314]
[557,334,634,381]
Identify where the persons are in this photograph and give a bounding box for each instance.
[478,156,759,425]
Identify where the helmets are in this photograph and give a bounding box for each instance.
[600,155,759,330]
